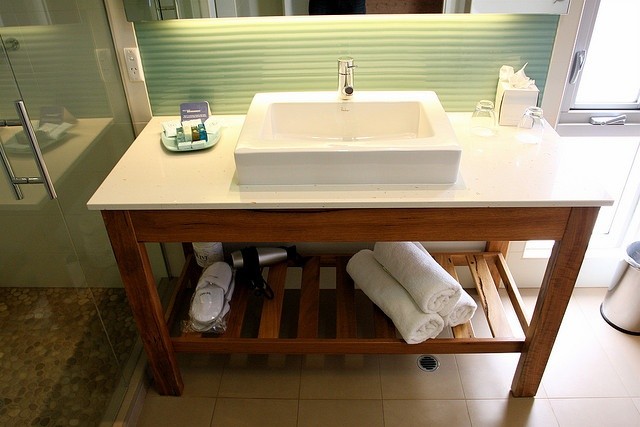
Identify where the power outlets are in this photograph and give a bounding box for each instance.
[95,48,117,84]
[122,48,144,85]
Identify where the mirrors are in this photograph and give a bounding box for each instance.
[125,1,568,21]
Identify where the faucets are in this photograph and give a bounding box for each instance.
[337,57,355,98]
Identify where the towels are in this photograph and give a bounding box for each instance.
[411,241,479,328]
[371,241,464,317]
[346,247,445,345]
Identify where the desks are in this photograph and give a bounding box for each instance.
[87,113,614,397]
[0,117,115,209]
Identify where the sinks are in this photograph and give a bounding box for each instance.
[233,89,461,151]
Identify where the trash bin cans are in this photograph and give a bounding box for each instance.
[600,241,640,336]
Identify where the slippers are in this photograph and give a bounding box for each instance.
[191,261,235,332]
